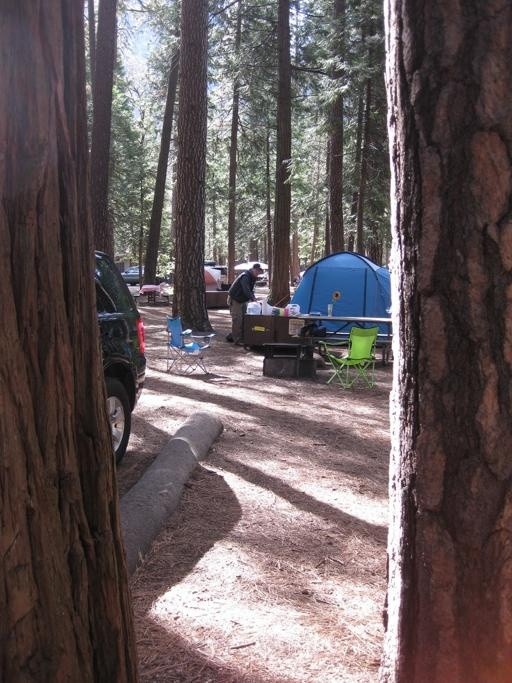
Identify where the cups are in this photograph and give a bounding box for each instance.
[327,304,333,317]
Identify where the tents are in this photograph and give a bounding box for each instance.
[288,250,393,344]
[204,265,223,290]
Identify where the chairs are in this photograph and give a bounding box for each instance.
[165,314,216,376]
[318,326,379,388]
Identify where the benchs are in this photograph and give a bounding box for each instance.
[303,330,393,364]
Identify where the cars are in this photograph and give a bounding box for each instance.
[95,251,146,465]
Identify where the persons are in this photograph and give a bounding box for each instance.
[226,263,265,345]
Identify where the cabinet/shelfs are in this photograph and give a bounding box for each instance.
[242,312,317,349]
[205,291,230,309]
[127,285,140,304]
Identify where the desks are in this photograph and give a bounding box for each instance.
[297,315,393,366]
[142,284,160,304]
